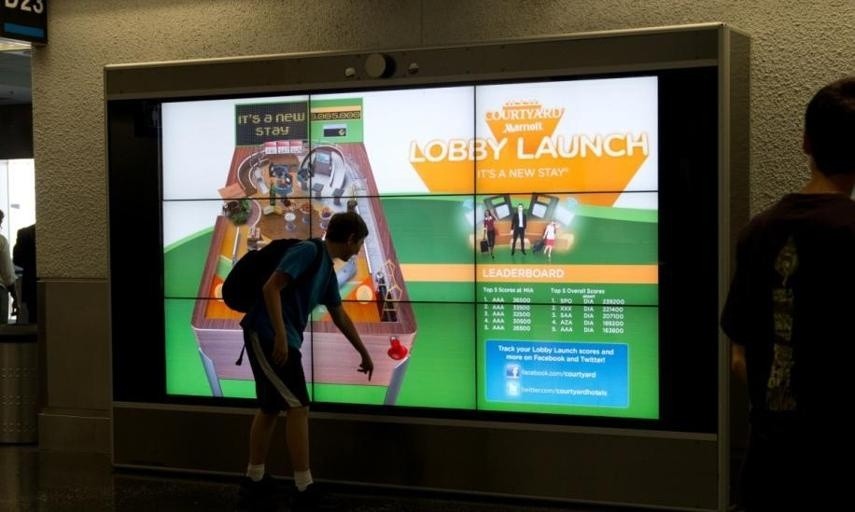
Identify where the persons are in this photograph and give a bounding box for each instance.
[0,209,35,332]
[483,209,496,259]
[542,220,560,260]
[510,203,527,255]
[722,77,854,511]
[222,212,374,498]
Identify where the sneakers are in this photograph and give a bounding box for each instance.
[238,474,293,497]
[289,481,336,512]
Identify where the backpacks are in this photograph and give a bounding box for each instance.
[222,238,323,316]
